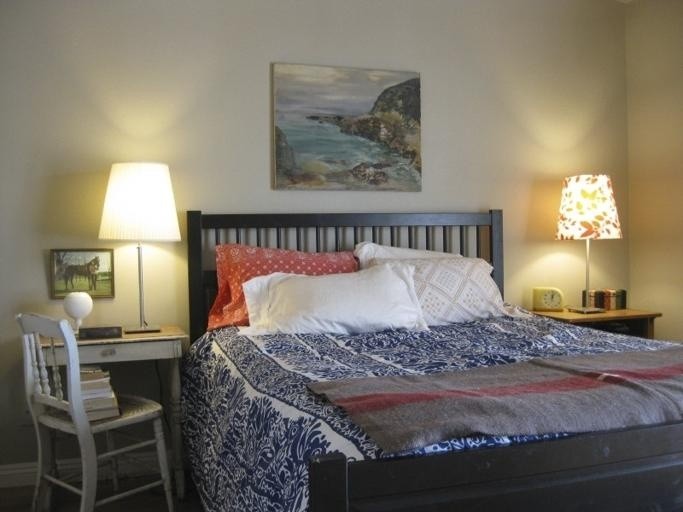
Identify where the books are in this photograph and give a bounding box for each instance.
[78,364,121,422]
[79,325,123,340]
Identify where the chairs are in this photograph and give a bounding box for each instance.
[14,311,176,512]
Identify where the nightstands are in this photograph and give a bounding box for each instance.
[40,325,190,504]
[529,307,662,340]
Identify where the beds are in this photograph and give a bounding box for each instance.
[179,208,682,511]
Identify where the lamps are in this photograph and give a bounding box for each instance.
[554,173,623,314]
[98,163,182,334]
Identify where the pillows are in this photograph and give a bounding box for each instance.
[369,257,515,328]
[354,239,462,270]
[237,259,431,336]
[207,244,359,332]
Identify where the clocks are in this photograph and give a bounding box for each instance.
[533,287,564,312]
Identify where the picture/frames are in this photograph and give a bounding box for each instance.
[49,248,115,299]
